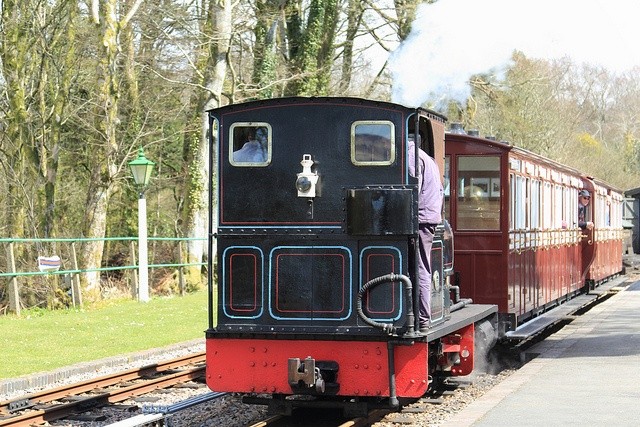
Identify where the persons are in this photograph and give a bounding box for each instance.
[578,191,594,230]
[406,134,443,330]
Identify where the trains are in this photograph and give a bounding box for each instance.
[203,96,640,414]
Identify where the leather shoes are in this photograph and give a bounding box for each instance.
[419,325,430,333]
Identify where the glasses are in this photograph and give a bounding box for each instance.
[582,196,590,200]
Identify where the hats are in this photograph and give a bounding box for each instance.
[579,190,591,198]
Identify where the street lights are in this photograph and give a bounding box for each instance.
[129,143,157,303]
